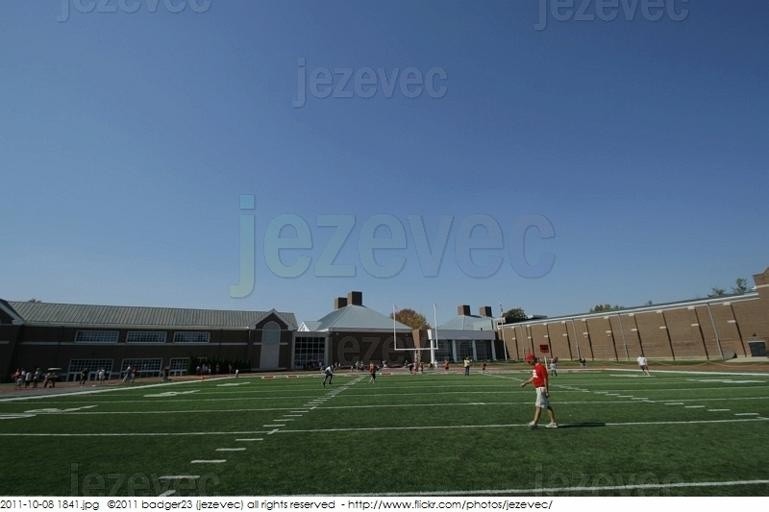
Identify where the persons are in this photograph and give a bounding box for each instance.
[321,364,335,387]
[464,356,472,375]
[79,366,106,384]
[520,353,558,428]
[11,366,61,388]
[159,363,170,380]
[636,352,650,376]
[122,363,138,384]
[234,367,242,378]
[550,357,559,376]
[366,362,379,384]
[192,359,233,375]
[480,361,489,374]
[313,358,452,372]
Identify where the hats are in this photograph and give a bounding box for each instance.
[525,353,536,361]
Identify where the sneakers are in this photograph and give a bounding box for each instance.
[545,422,558,428]
[529,421,537,427]
